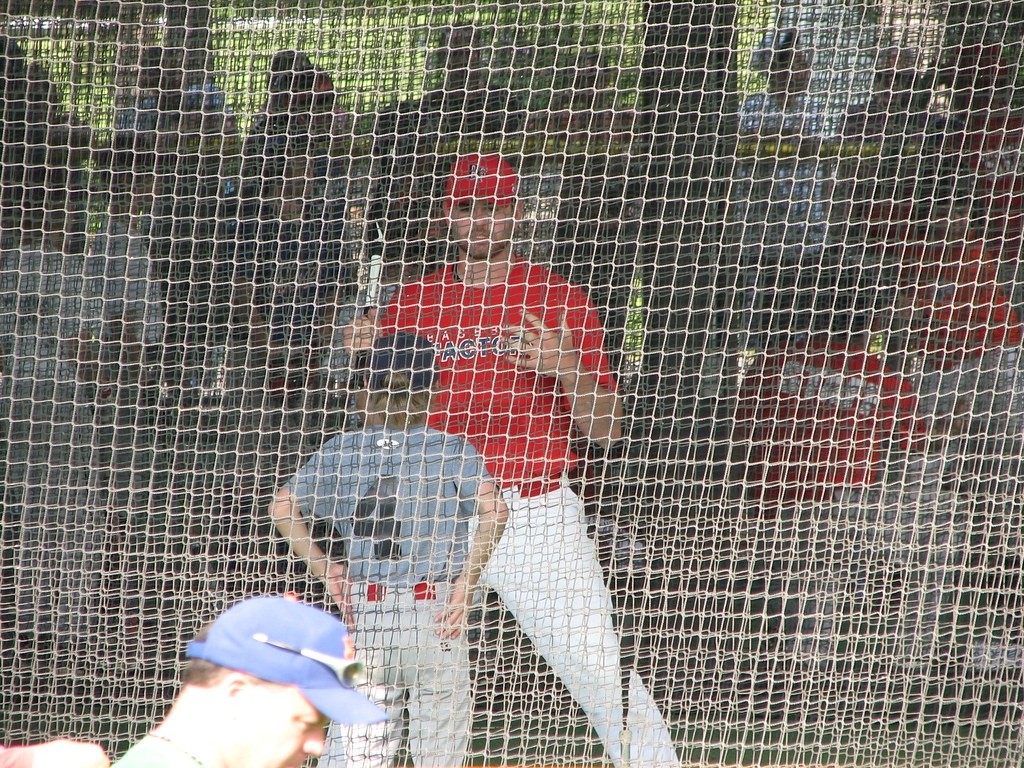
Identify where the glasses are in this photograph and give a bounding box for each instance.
[253,632,364,688]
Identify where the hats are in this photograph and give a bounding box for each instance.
[365,332,439,390]
[185,597,388,725]
[444,154,518,208]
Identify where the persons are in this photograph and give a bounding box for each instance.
[647,10,1023,689]
[265,332,509,768]
[104,593,393,767]
[339,153,683,768]
[0,10,528,640]
